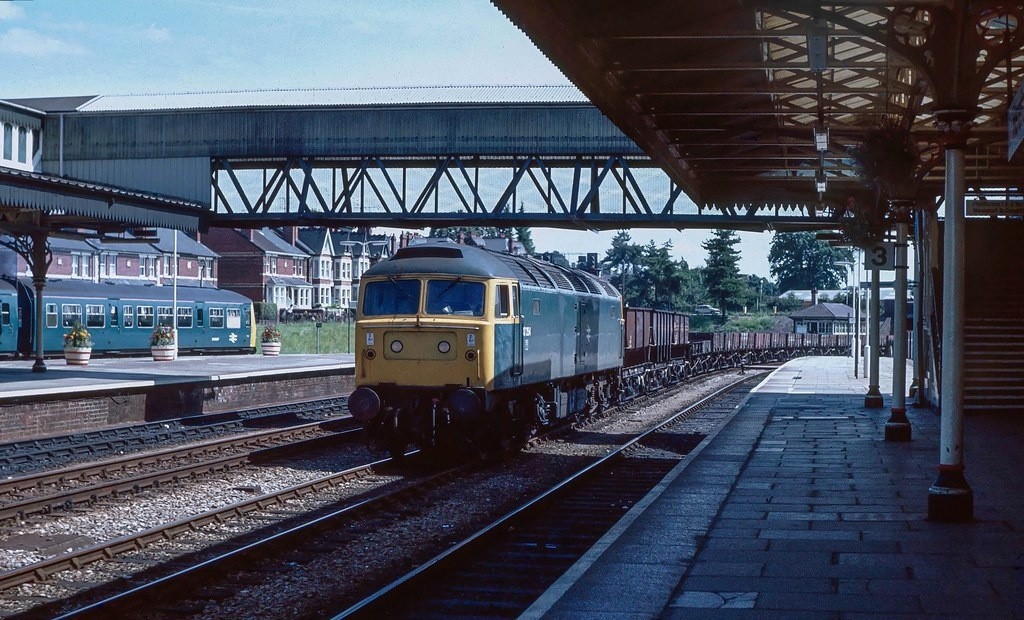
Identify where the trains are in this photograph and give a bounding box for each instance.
[344,233,893,463]
[0,272,257,357]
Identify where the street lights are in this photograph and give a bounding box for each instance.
[835,245,871,380]
[832,261,864,357]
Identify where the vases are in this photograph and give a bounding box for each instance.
[64,347,92,365]
[261,342,281,356]
[151,345,174,361]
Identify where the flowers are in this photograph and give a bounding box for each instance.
[63,320,93,347]
[261,323,280,341]
[149,325,174,344]
